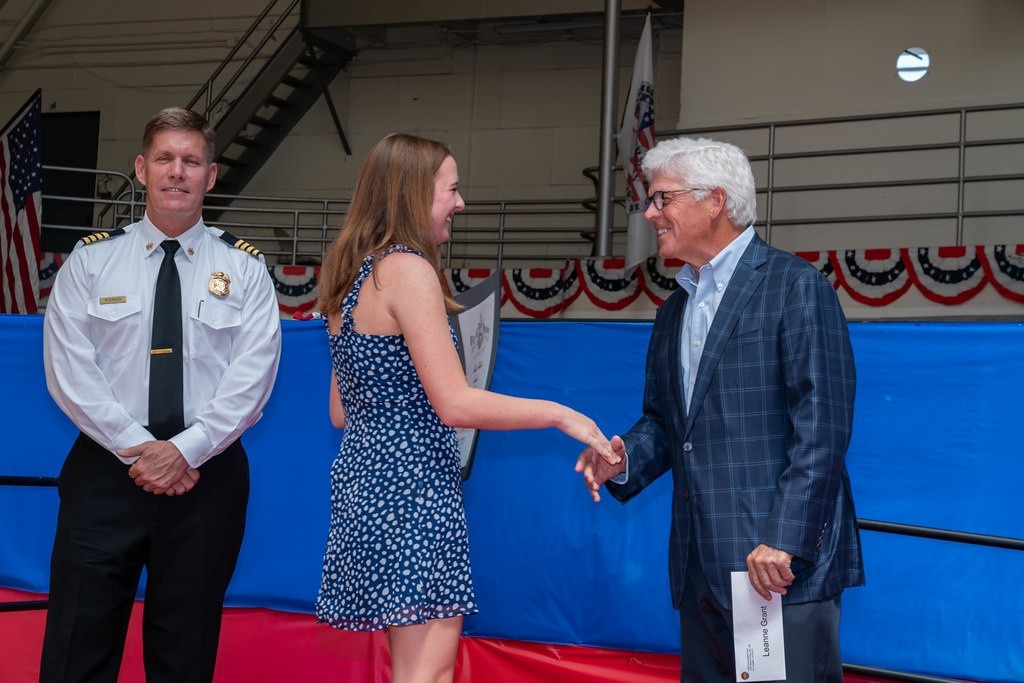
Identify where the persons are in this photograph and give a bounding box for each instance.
[314,134,622,683]
[574,138,865,683]
[39,106,281,683]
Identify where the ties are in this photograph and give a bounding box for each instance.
[147,239,186,441]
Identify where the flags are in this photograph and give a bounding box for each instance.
[0,97,41,315]
[618,12,661,280]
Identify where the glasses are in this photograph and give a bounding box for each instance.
[644,187,706,210]
